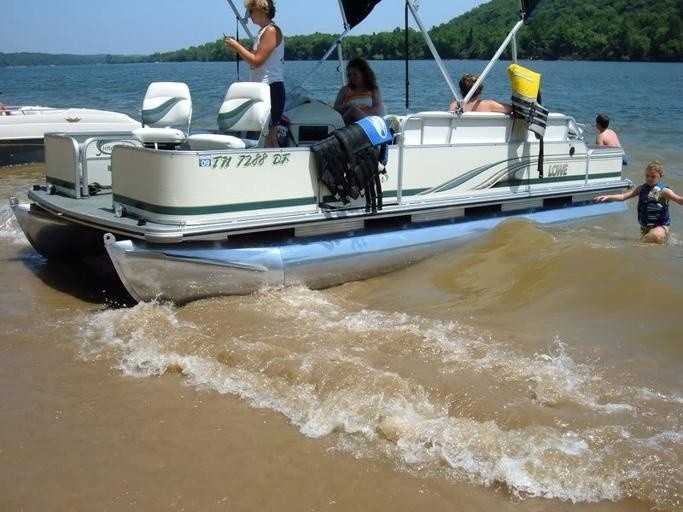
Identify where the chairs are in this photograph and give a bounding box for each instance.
[132,79,193,151]
[186,81,272,152]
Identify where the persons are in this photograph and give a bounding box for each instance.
[223,1,284,149]
[0,91,10,116]
[592,161,682,243]
[595,114,628,165]
[333,58,386,127]
[448,74,514,114]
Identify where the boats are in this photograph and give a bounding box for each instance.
[8,0,633,305]
[0,106,150,162]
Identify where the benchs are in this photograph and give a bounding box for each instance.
[396,110,579,145]
